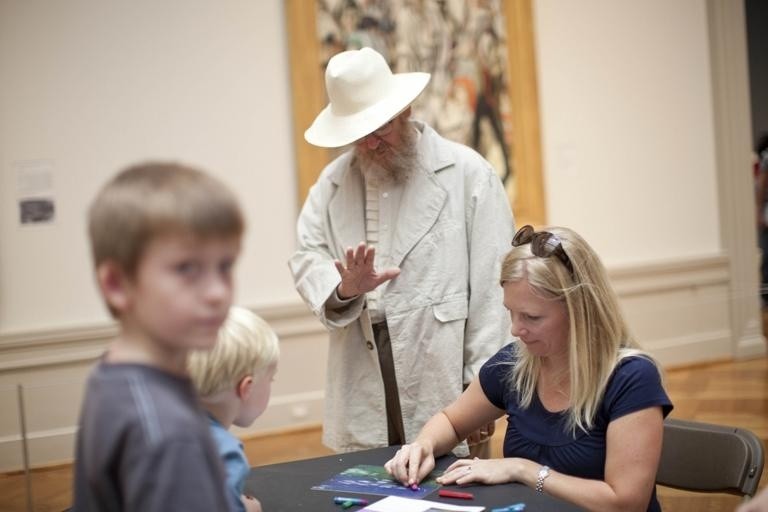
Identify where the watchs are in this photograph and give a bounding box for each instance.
[535,463,550,495]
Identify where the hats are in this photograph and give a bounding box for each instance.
[304,47,432,148]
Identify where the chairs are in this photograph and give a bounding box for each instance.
[655,417,764,512]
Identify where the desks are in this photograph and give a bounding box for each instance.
[242,445,583,512]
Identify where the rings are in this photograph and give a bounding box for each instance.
[467,466,474,471]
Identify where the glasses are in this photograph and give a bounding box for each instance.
[511,225,572,272]
[355,116,398,144]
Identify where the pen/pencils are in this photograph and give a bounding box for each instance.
[439,489,474,499]
[330,494,366,505]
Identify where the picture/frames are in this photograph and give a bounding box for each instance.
[282,0,547,233]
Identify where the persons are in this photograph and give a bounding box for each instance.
[190,307,282,510]
[285,43,515,459]
[382,224,673,512]
[732,485,768,511]
[73,158,244,512]
